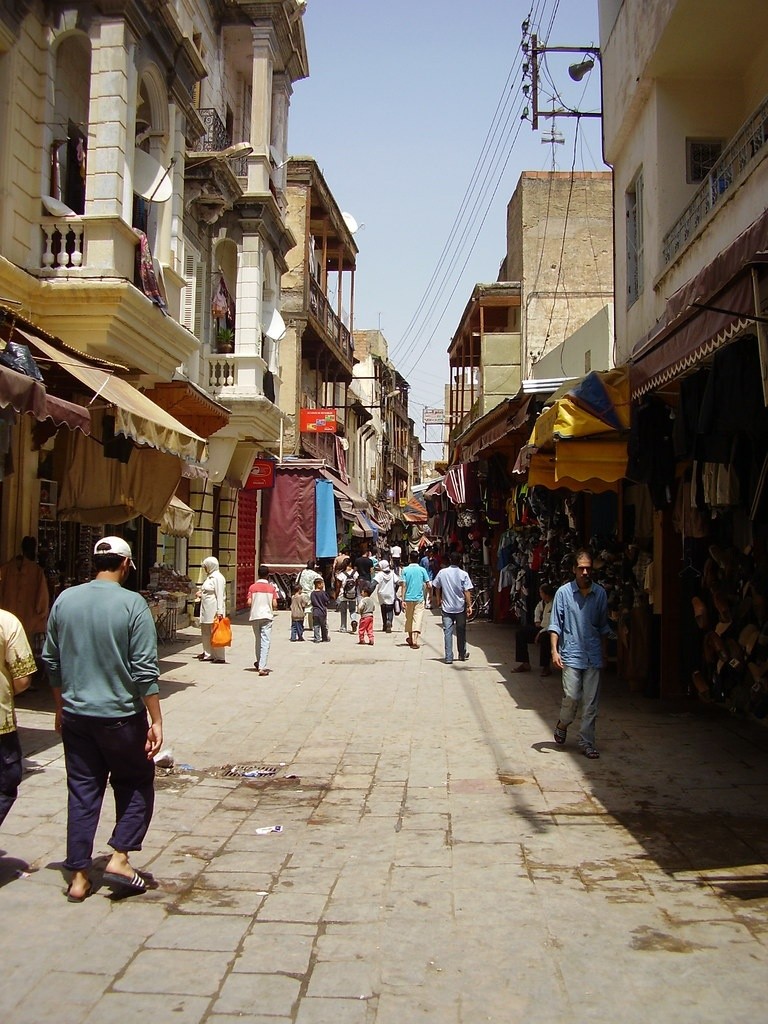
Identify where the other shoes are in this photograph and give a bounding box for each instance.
[352,621,357,632]
[386,620,391,633]
[459,653,469,660]
[202,658,225,663]
[259,671,268,676]
[510,664,532,673]
[539,667,552,677]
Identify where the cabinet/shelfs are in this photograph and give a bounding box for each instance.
[39,478,57,522]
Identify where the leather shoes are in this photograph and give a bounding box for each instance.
[690,543,768,720]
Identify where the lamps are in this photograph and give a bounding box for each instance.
[372,390,400,404]
[568,54,595,81]
[184,143,253,171]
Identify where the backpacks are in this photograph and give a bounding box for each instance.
[342,566,358,599]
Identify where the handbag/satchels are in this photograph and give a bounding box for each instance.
[210,615,232,647]
[394,592,402,615]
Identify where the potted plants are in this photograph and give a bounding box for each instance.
[216,327,234,353]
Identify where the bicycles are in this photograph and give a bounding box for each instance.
[466,585,494,622]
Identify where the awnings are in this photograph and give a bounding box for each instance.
[0,322,211,531]
[319,462,467,542]
[512,363,630,493]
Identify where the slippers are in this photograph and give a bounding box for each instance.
[553,719,567,743]
[578,745,600,758]
[103,870,147,892]
[68,878,98,904]
[406,638,420,649]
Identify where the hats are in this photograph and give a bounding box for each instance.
[93,536,137,572]
[449,552,459,561]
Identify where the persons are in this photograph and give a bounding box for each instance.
[196,556,226,663]
[511,583,554,678]
[318,541,449,633]
[289,585,307,642]
[311,578,331,643]
[432,552,474,664]
[295,559,325,631]
[548,551,617,758]
[364,560,403,633]
[0,536,49,633]
[246,566,278,676]
[40,536,163,903]
[0,608,39,827]
[356,587,375,645]
[401,551,432,649]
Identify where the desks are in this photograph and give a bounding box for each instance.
[154,607,181,648]
